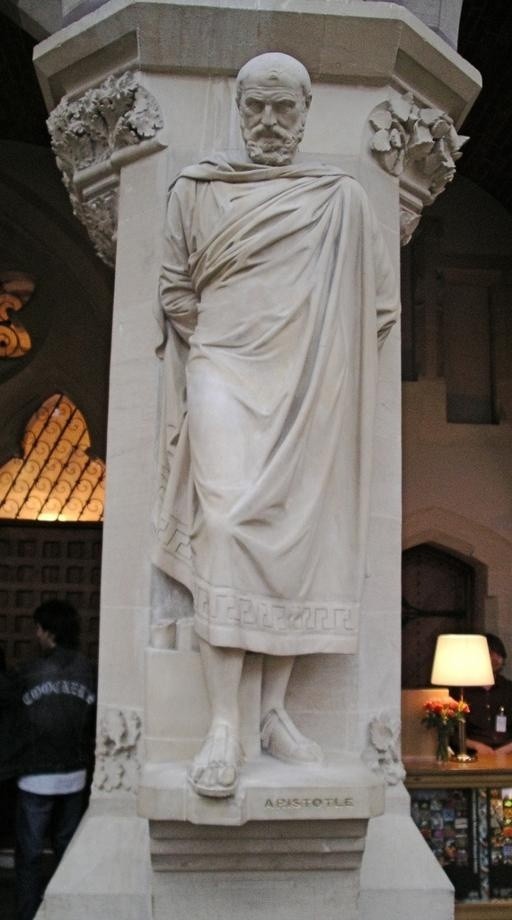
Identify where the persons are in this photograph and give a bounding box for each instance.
[456,632,512,749]
[155,47,400,797]
[7,598,97,920]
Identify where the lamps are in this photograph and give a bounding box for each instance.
[429,631,494,760]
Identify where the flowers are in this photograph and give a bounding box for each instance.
[417,699,470,760]
